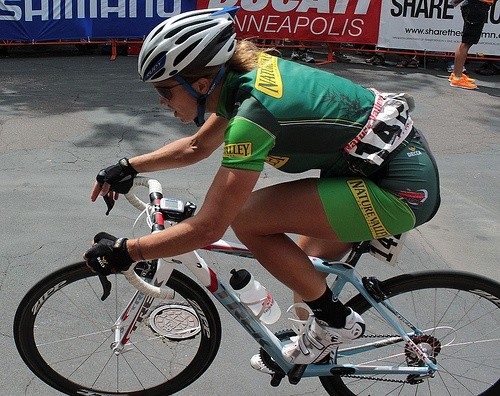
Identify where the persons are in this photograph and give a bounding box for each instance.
[333,43,351,63]
[446,57,467,74]
[397,49,420,68]
[82,5,441,374]
[291,40,314,63]
[449,0,493,89]
[366,46,385,63]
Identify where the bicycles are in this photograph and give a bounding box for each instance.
[12,174,500,396]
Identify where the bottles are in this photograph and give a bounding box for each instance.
[228,268,281,325]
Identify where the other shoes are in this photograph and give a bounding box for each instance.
[447,65,454,72]
[397,59,419,68]
[333,52,350,62]
[461,65,467,74]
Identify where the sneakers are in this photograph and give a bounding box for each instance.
[250,354,275,374]
[450,77,477,89]
[282,307,366,364]
[449,72,475,83]
[300,48,315,63]
[290,48,300,60]
[366,54,385,64]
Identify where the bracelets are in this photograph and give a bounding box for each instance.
[137,238,145,260]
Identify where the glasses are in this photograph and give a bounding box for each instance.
[154,74,210,101]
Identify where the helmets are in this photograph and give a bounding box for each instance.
[138,7,240,82]
